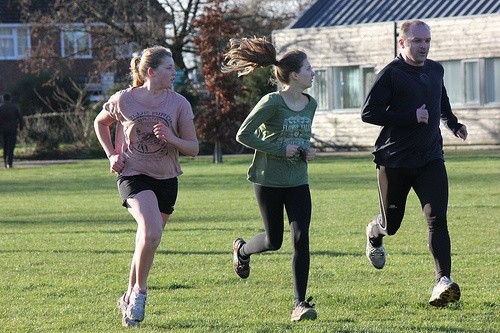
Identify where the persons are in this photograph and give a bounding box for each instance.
[360,19,468,307]
[0,93,24,168]
[221,34,317,323]
[94,45,200,327]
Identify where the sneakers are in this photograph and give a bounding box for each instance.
[366,221,386,269]
[117,291,140,328]
[127,284,148,322]
[232,237,251,279]
[429,272,461,306]
[290,296,318,322]
